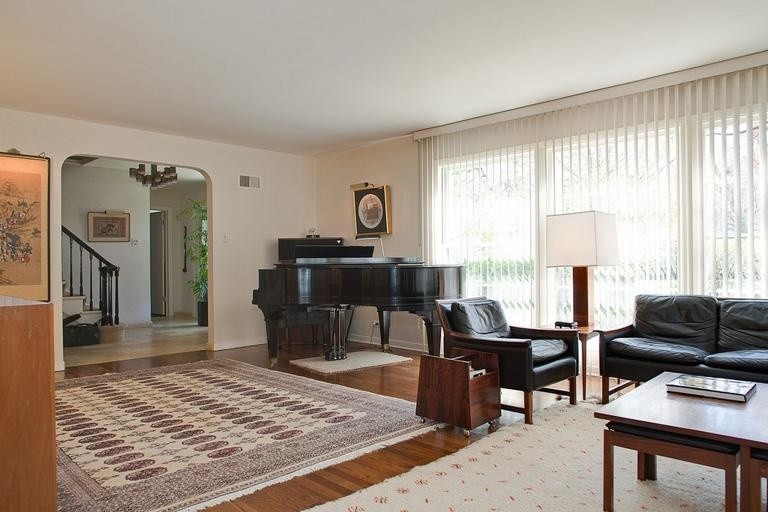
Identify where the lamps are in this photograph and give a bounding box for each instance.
[355,234,385,257]
[127,162,177,190]
[544,211,617,327]
[348,182,374,189]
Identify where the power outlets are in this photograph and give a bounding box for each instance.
[373,320,379,327]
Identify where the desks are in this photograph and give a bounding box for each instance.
[594,369,768,511]
[541,322,613,401]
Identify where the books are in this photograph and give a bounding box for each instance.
[665,372,758,402]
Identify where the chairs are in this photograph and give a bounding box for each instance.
[437,298,578,426]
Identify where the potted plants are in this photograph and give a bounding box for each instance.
[179,201,209,327]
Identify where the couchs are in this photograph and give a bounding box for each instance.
[592,290,766,406]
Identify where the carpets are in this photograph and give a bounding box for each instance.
[54,358,453,512]
[299,387,767,512]
[290,344,414,377]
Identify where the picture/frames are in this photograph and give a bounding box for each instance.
[86,211,130,243]
[351,184,390,236]
[0,149,51,304]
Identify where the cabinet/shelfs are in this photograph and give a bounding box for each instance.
[0,294,58,512]
[277,237,343,342]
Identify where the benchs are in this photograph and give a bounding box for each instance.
[279,313,330,351]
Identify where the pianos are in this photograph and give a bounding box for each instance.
[252,245,466,361]
